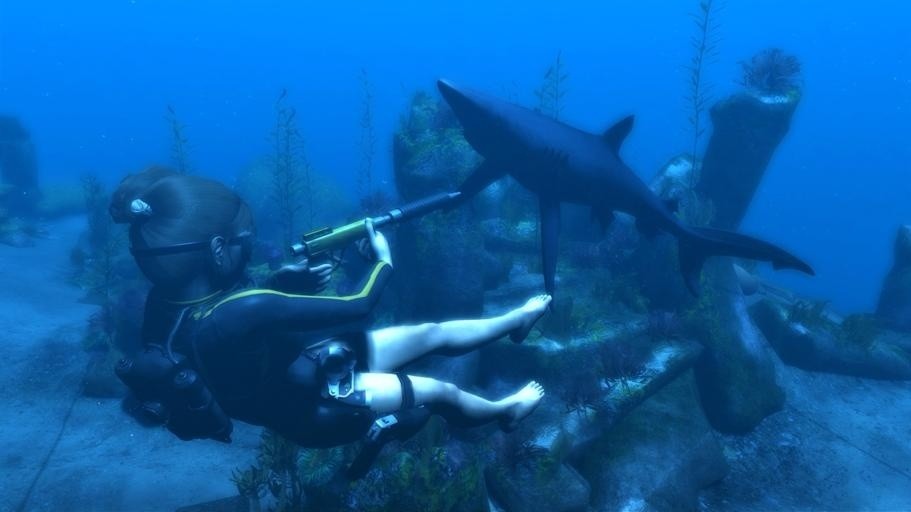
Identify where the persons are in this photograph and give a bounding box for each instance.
[108,164,556,447]
